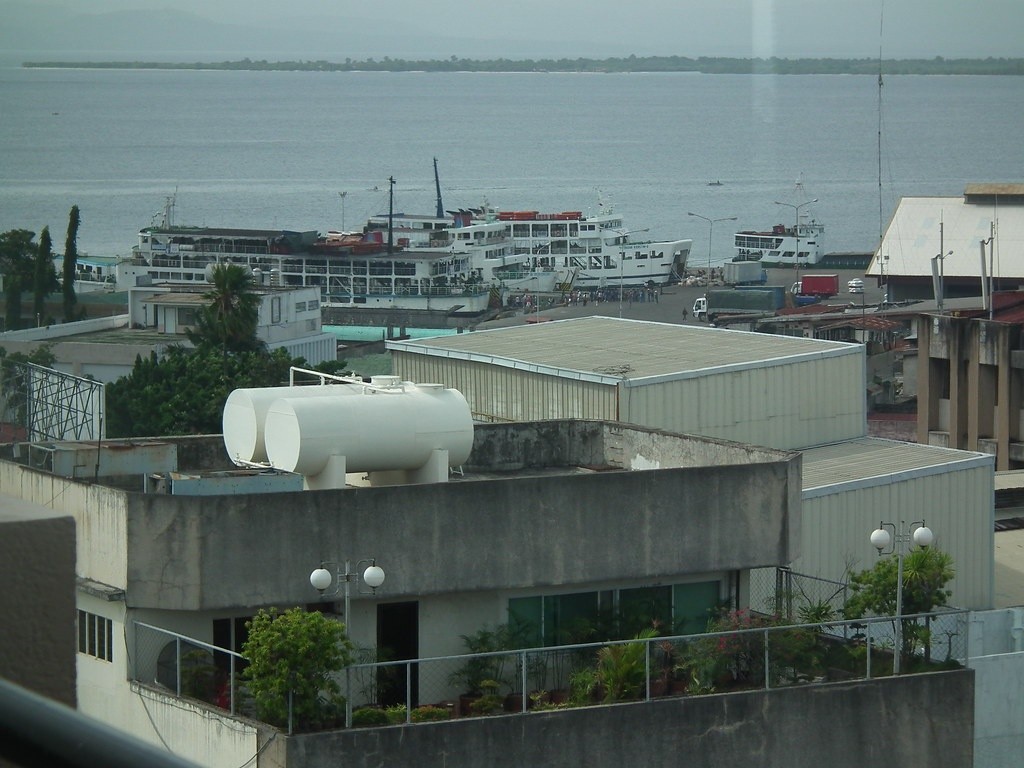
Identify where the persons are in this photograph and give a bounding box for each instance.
[328,259,723,322]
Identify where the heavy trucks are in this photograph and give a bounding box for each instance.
[723,261,768,286]
[733,285,785,310]
[692,289,777,324]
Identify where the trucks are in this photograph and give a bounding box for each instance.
[792,274,839,299]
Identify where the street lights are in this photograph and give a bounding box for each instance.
[774,198,819,282]
[869,519,934,675]
[598,227,650,319]
[310,558,387,732]
[687,212,737,288]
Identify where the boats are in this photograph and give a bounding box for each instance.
[54,145,695,315]
[707,180,724,186]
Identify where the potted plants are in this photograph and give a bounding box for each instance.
[171,533,962,735]
